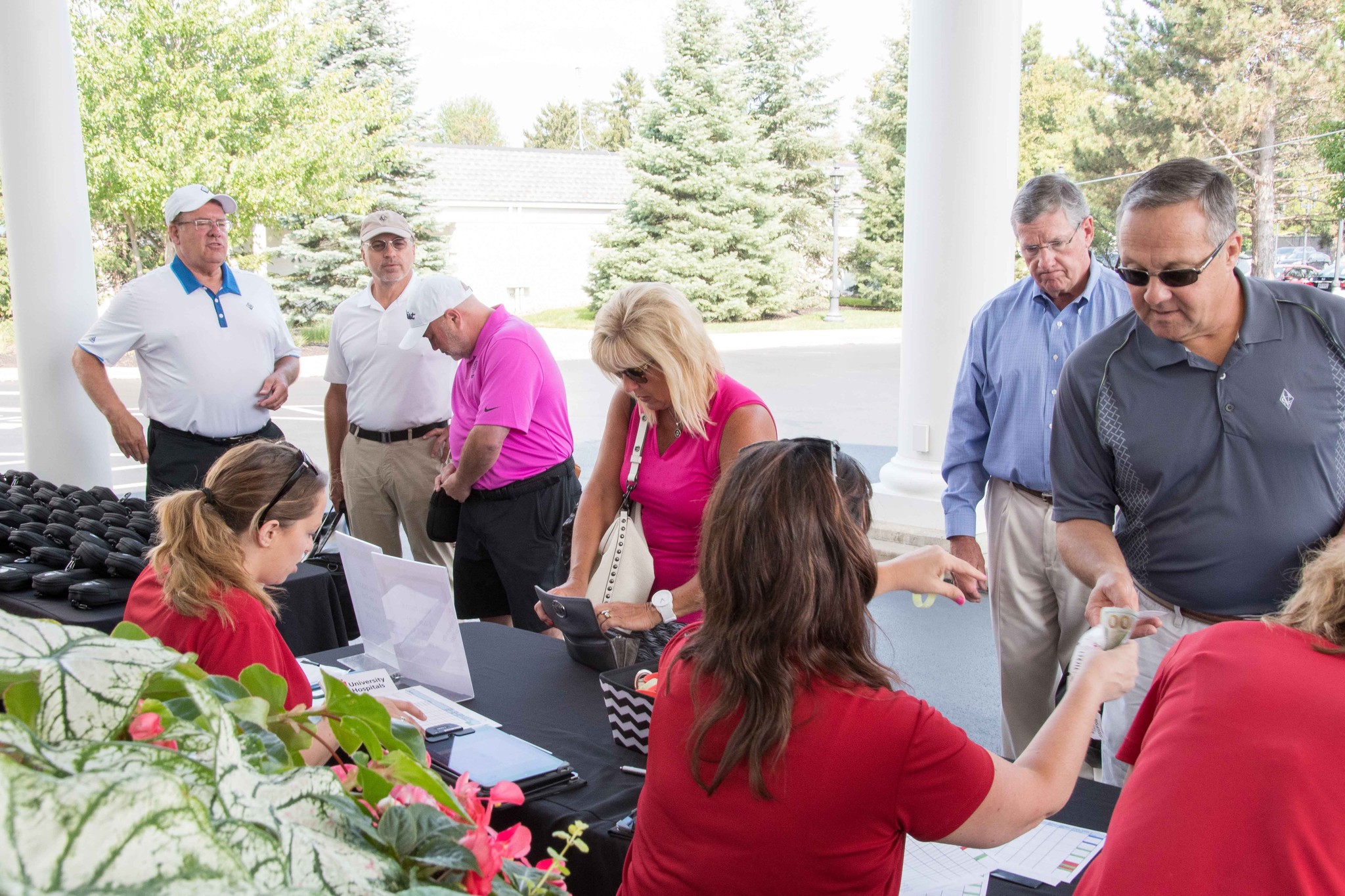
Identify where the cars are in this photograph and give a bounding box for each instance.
[1235,245,1345,298]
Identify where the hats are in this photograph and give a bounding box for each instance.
[164,183,238,227]
[397,275,473,351]
[360,210,416,241]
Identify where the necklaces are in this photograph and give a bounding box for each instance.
[668,407,682,439]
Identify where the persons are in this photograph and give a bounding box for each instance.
[941,175,1135,760]
[534,283,780,650]
[1050,157,1344,788]
[616,434,1140,896]
[323,212,457,570]
[405,276,582,643]
[123,440,426,825]
[1074,525,1345,896]
[71,185,300,501]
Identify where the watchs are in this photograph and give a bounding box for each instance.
[651,590,677,624]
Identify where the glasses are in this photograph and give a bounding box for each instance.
[256,440,319,531]
[176,219,234,232]
[1016,222,1082,258]
[738,437,840,481]
[614,359,656,383]
[1114,227,1237,287]
[362,238,411,252]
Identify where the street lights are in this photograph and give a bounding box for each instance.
[821,158,845,323]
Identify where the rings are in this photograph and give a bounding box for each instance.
[605,612,612,619]
[400,715,407,722]
[279,404,281,407]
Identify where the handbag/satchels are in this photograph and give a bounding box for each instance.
[304,500,361,641]
[584,412,656,636]
[426,487,461,542]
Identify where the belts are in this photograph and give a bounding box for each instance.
[150,418,272,445]
[469,455,575,501]
[1131,575,1245,625]
[349,419,448,442]
[1003,479,1053,505]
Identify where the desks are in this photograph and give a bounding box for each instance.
[0,543,1122,896]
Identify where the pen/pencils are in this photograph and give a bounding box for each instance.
[619,766,647,777]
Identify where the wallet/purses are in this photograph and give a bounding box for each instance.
[533,584,641,670]
